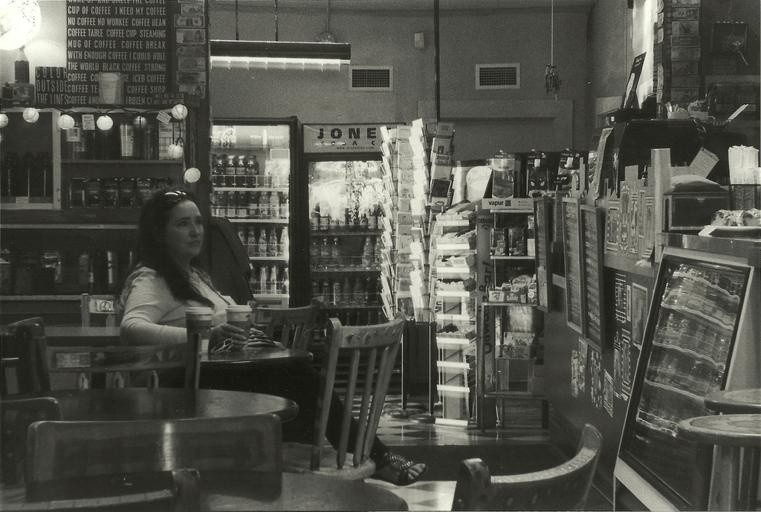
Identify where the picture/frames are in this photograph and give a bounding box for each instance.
[578,202,605,355]
[560,196,582,335]
[531,196,552,314]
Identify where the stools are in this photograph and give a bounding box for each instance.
[676,413,761,512]
[704,386,761,511]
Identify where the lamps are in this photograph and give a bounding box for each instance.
[0,0,44,52]
[207,0,351,65]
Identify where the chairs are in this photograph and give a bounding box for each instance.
[279,309,407,487]
[1,316,50,394]
[445,424,603,512]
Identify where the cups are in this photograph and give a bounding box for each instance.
[100,73,120,104]
[223,303,253,336]
[184,306,214,354]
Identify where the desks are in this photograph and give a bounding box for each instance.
[54,342,292,379]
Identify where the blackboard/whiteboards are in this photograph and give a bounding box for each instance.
[561,197,605,355]
[534,195,551,313]
[612,246,755,512]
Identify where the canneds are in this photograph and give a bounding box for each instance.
[68,177,169,208]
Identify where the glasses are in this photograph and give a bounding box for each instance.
[162,189,189,204]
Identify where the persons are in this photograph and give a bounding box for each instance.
[113,186,428,486]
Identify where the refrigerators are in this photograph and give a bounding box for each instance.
[300,121,410,397]
[212,115,297,312]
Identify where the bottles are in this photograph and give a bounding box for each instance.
[68,176,171,207]
[13,47,29,83]
[235,221,289,259]
[250,263,289,296]
[101,250,118,291]
[309,191,386,233]
[214,190,290,221]
[311,276,380,302]
[310,236,385,270]
[213,152,260,188]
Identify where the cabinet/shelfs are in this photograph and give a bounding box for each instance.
[211,175,290,297]
[0,103,195,326]
[307,226,385,310]
